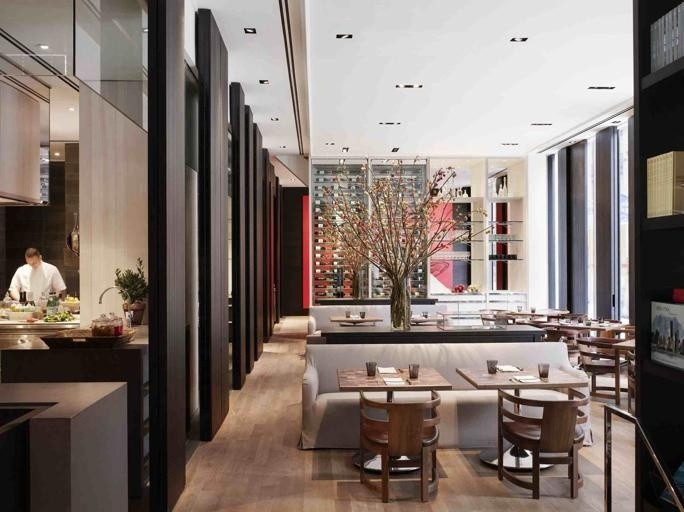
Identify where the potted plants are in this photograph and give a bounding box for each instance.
[116,257,148,326]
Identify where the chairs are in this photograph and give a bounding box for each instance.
[498,390,591,509]
[578,339,628,405]
[358,392,443,504]
[481,310,502,324]
[627,349,636,414]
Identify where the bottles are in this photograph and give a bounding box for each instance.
[91,313,115,337]
[54,291,58,315]
[122,302,131,331]
[110,312,124,335]
[47,291,56,318]
[58,294,64,313]
[39,291,47,313]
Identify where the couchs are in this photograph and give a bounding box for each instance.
[302,343,590,450]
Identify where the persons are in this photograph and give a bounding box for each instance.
[6,247,67,306]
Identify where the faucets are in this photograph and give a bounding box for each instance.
[98,286,132,329]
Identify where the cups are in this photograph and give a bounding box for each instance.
[367,361,376,376]
[518,306,522,312]
[360,312,365,318]
[409,363,419,379]
[346,311,351,318]
[565,315,571,323]
[599,315,604,323]
[578,315,583,323]
[586,318,592,326]
[531,307,536,313]
[423,312,428,319]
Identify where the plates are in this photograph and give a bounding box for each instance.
[487,360,498,373]
[377,366,396,374]
[41,327,135,347]
[383,377,404,384]
[539,364,550,378]
[497,365,520,372]
[514,375,541,383]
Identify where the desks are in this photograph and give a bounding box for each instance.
[545,321,626,356]
[331,315,382,328]
[337,367,450,472]
[457,366,588,469]
[412,313,448,324]
[511,308,569,324]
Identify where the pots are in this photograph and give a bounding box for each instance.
[66,213,79,256]
